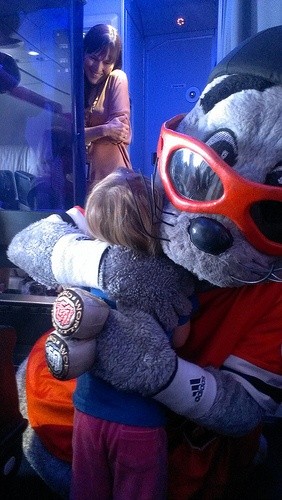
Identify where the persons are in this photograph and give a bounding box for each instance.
[71,167,202,500]
[83,25,132,201]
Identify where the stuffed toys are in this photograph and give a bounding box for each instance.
[5,25,281,500]
